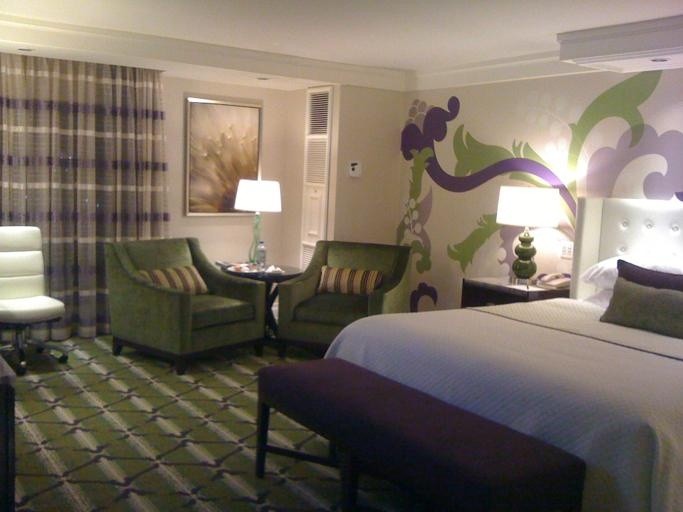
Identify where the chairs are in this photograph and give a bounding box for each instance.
[276,239,412,358]
[0,226,70,375]
[104,236,267,375]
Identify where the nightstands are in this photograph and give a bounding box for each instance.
[461,279,568,309]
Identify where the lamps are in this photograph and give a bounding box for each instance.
[496,184,563,283]
[234,178,283,263]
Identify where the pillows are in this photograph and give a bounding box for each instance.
[617,260,683,290]
[583,291,614,308]
[600,277,683,337]
[316,264,383,295]
[139,264,210,295]
[581,256,683,289]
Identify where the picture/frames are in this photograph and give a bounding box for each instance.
[185,97,264,217]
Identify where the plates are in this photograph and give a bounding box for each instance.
[227,263,267,275]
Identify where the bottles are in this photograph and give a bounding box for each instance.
[255,241,266,269]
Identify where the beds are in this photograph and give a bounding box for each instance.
[322,195,683,511]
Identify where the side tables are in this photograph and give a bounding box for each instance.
[222,260,304,336]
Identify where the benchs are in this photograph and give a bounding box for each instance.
[257,358,587,510]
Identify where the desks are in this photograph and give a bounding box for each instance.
[0,358,15,511]
